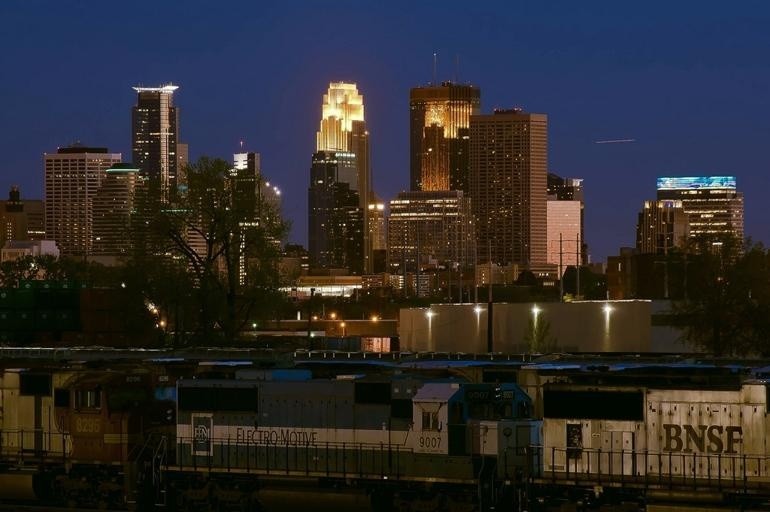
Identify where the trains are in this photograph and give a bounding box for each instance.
[0,355,770,512]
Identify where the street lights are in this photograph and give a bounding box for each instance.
[341,322,346,337]
[253,323,257,335]
